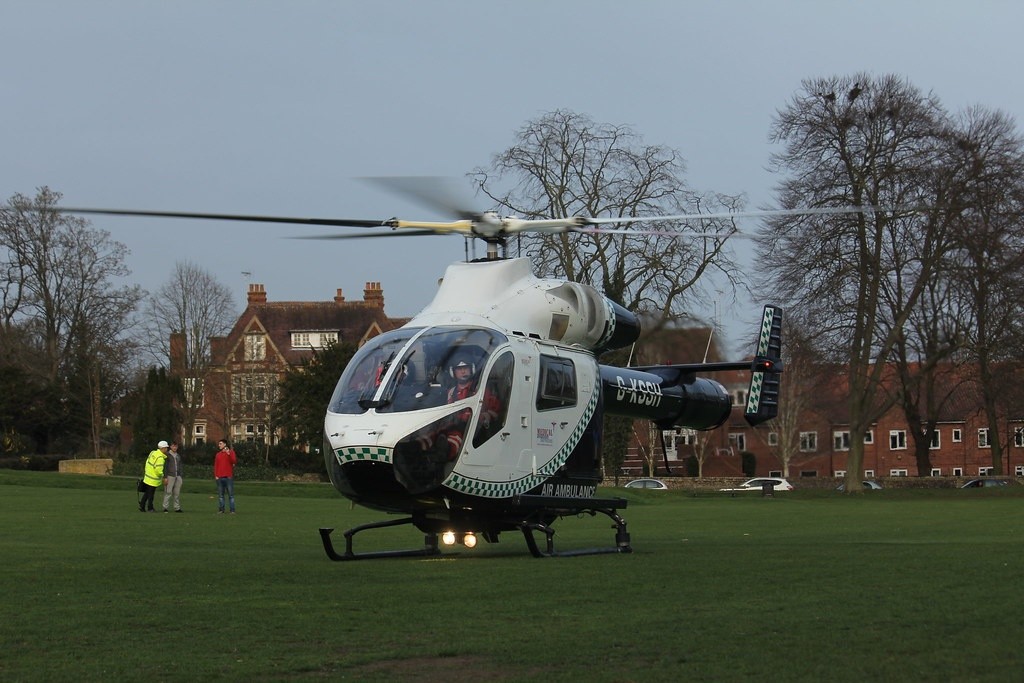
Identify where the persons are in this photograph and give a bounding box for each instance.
[140,441,170,512]
[378,355,504,486]
[213,439,238,515]
[162,442,184,514]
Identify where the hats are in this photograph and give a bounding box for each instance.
[158,441,169,448]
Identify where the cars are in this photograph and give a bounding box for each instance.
[836,480,883,491]
[623,478,668,490]
[960,477,1024,489]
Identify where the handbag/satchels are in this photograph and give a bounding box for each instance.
[138,479,145,492]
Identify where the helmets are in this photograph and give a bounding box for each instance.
[449,353,476,379]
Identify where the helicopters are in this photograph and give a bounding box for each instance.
[1,173,962,563]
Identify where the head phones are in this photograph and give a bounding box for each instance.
[449,362,476,379]
[401,364,409,380]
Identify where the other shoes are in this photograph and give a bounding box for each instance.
[164,509,168,513]
[148,508,156,512]
[175,509,183,513]
[217,510,224,515]
[435,434,448,463]
[137,506,146,512]
[230,511,235,515]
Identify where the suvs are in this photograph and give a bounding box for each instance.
[720,476,793,491]
